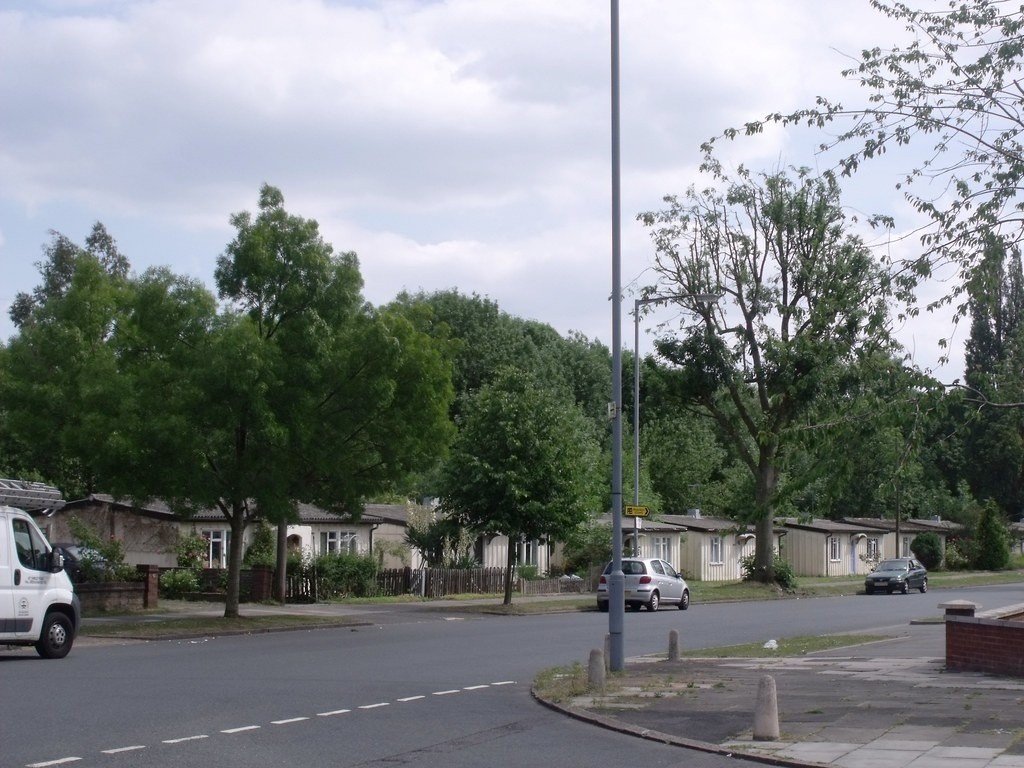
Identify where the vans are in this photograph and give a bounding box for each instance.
[0,505,83,659]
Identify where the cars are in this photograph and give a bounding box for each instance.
[865,557,929,594]
[49,543,115,595]
[596,556,690,611]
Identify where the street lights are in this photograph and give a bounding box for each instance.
[629,293,725,558]
